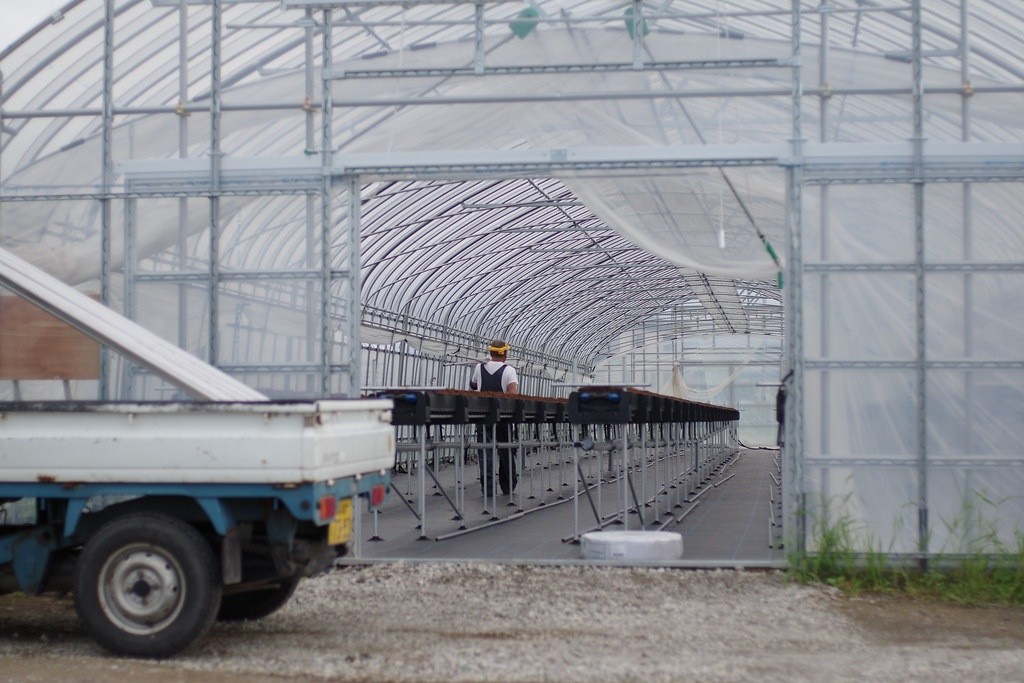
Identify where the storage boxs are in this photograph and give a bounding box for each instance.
[578,530,684,561]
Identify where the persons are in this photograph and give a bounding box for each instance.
[469,340,518,496]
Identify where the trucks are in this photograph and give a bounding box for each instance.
[0,248,397,658]
